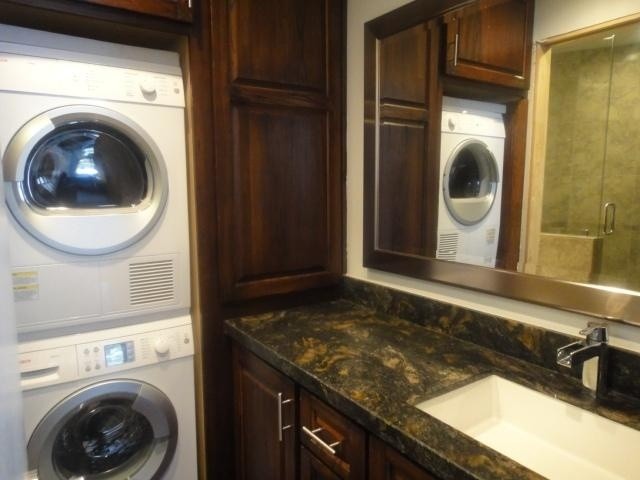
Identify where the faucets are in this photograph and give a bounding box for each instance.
[553,322,609,406]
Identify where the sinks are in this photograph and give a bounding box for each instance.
[414,372,640,480]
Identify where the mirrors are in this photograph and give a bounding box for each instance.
[363,0,640,326]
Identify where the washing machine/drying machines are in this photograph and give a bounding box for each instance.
[0,315,198,480]
[0,24,192,333]
[436,96,508,269]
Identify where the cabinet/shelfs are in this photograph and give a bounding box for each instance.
[211,0,343,306]
[379,15,440,258]
[299,387,368,480]
[229,340,300,480]
[0,0,193,47]
[445,0,534,90]
[369,434,437,480]
